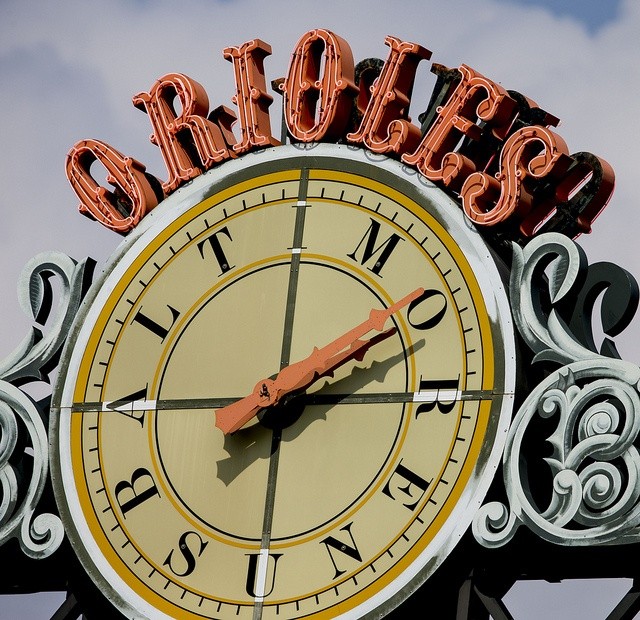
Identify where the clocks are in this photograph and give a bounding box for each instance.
[42,142,520,614]
